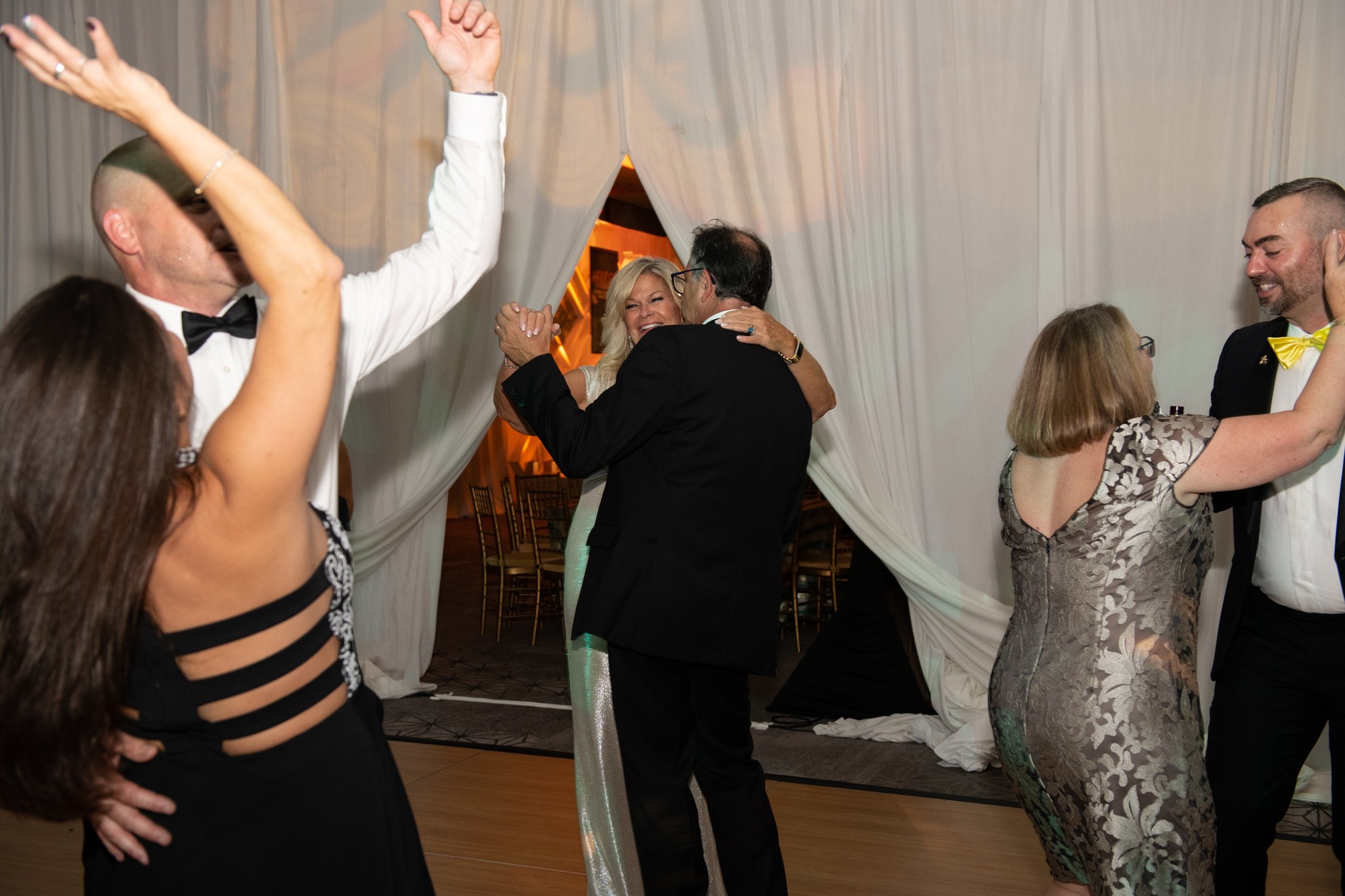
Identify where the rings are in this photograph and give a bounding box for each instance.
[494,325,501,332]
[54,61,65,77]
[748,324,756,335]
[528,309,537,313]
[76,57,89,74]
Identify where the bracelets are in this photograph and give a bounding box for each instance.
[194,149,239,196]
[503,354,520,369]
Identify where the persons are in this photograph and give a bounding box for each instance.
[1202,177,1344,896]
[0,8,442,896]
[492,256,836,896]
[988,226,1345,896]
[492,218,814,896]
[82,0,509,871]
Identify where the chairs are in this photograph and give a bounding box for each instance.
[781,532,803,654]
[526,486,568,652]
[796,509,857,631]
[501,476,562,628]
[470,482,565,644]
[516,474,567,544]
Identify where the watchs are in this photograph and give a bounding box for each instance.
[778,333,804,365]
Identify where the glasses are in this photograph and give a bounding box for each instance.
[1136,336,1156,358]
[670,267,717,295]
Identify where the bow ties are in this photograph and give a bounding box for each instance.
[1267,325,1334,370]
[181,294,259,355]
